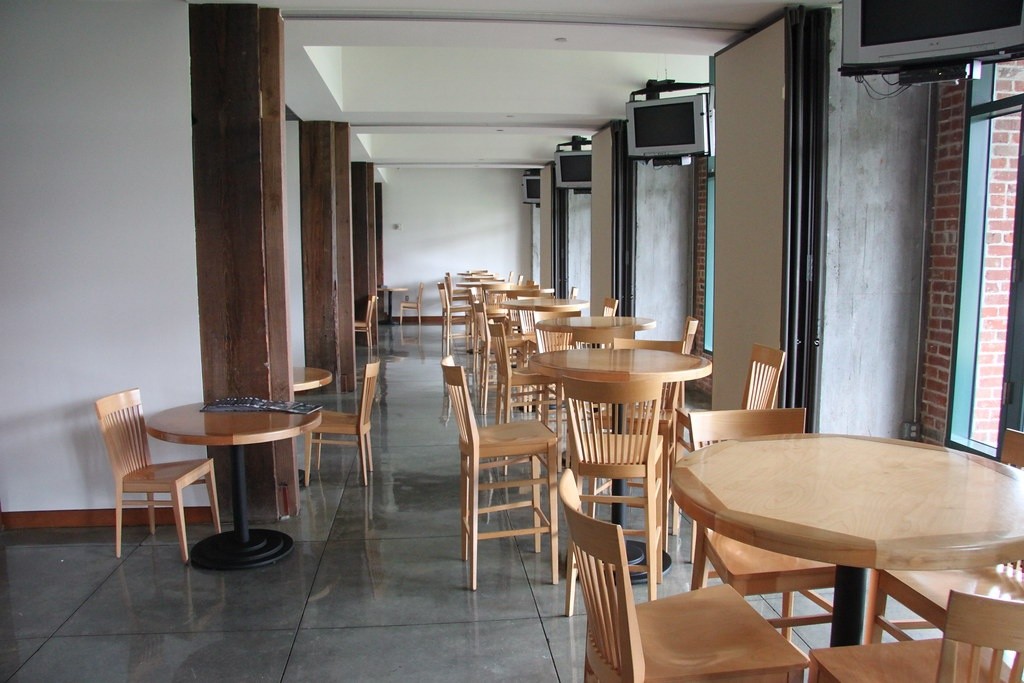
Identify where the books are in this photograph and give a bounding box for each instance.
[200,396,323,414]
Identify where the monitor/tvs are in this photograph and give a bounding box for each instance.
[554,150,592,188]
[626,94,708,157]
[842,0,1024,66]
[522,176,541,204]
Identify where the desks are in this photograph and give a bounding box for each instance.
[501,299,591,412]
[672,434,1024,647]
[377,288,408,325]
[291,366,332,487]
[457,272,487,317]
[456,282,513,354]
[146,402,322,569]
[486,288,554,368]
[462,276,494,334]
[529,349,713,585]
[535,316,656,413]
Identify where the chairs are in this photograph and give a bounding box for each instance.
[95,388,221,566]
[437,272,1024,683]
[355,295,375,347]
[400,283,425,326]
[304,359,380,486]
